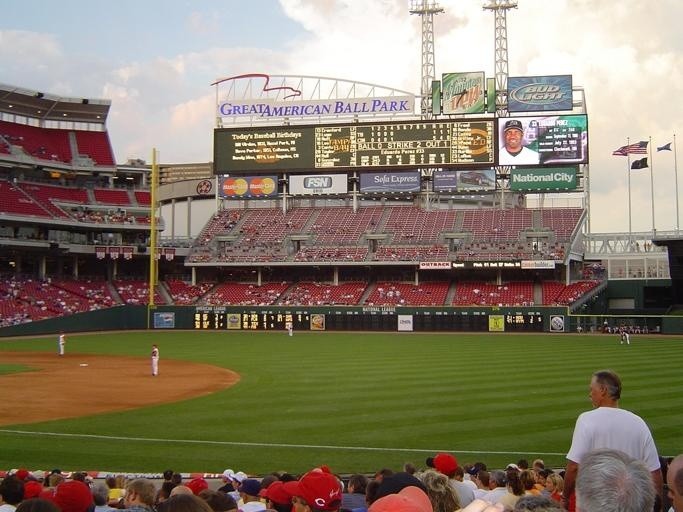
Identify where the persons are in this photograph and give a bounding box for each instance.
[499,120,539,164]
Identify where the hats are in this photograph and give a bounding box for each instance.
[184,477,208,496]
[282,472,343,510]
[24,481,42,499]
[467,462,486,474]
[426,454,458,475]
[260,481,291,505]
[239,480,260,496]
[39,481,93,512]
[223,469,248,483]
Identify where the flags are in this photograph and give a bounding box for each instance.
[631,158,648,169]
[657,143,671,151]
[613,146,627,156]
[625,142,648,154]
